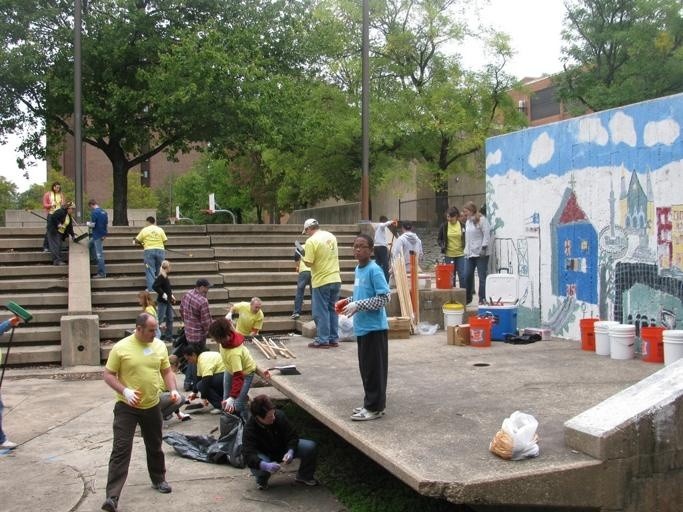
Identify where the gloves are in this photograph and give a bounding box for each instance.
[282,448,294,465]
[222,397,235,413]
[340,302,357,318]
[335,299,347,314]
[478,248,485,259]
[8,317,20,327]
[259,462,280,474]
[123,388,141,407]
[170,390,181,406]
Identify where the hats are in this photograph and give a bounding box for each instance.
[301,218,318,235]
[195,279,213,288]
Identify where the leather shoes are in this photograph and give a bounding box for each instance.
[307,342,329,349]
[329,342,338,347]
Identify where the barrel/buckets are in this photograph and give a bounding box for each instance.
[468,315,494,347]
[435,264,455,289]
[662,328,683,367]
[579,318,599,351]
[608,324,636,359]
[640,326,667,363]
[442,300,465,331]
[593,320,620,356]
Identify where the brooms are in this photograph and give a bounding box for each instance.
[30,210,89,243]
[0,301,34,387]
[243,332,297,360]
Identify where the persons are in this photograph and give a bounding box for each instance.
[2,317,21,452]
[137,292,161,340]
[42,182,64,251]
[333,235,392,420]
[152,260,177,341]
[46,201,76,263]
[291,201,491,349]
[180,276,266,422]
[134,216,168,290]
[241,394,319,489]
[98,313,182,510]
[86,198,107,279]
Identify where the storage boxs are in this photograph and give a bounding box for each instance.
[386,316,411,340]
[476,273,520,341]
[446,324,470,346]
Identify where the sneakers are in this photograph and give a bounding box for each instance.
[101,499,117,512]
[0,440,17,449]
[210,409,221,414]
[54,260,65,265]
[349,408,381,421]
[352,406,385,416]
[294,478,316,486]
[290,313,299,319]
[93,274,106,278]
[150,481,172,493]
[256,482,268,491]
